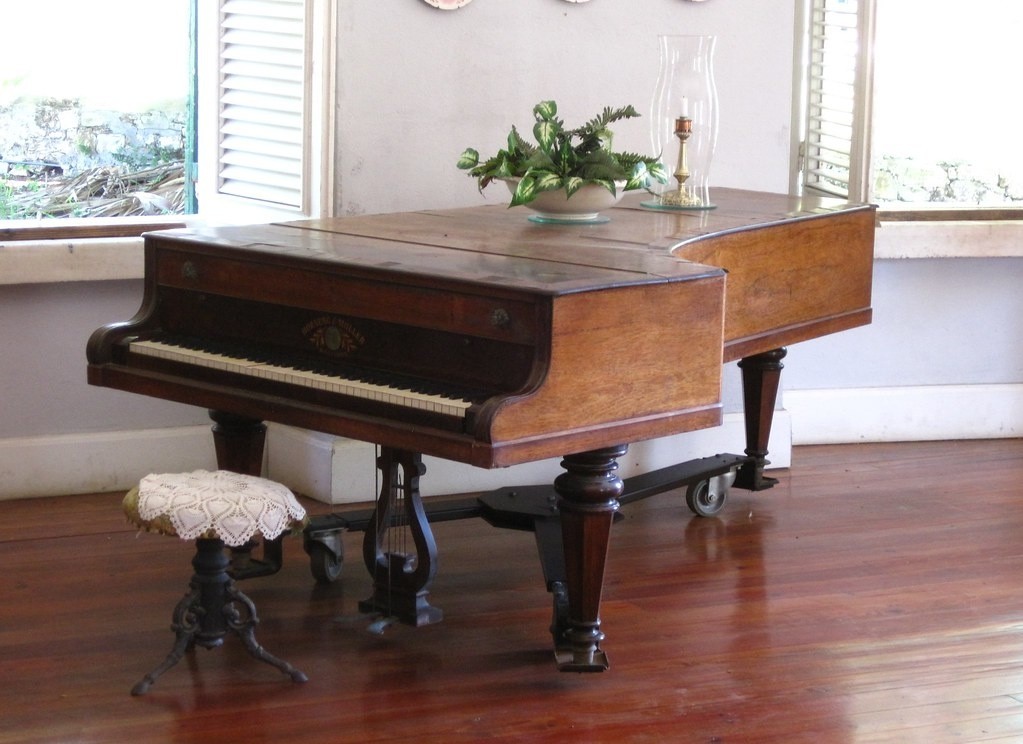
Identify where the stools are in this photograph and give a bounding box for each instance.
[119,469,310,696]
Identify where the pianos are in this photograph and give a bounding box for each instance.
[83,186,880,674]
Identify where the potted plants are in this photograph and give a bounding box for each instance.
[456,100,669,221]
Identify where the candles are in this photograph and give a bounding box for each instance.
[679,93,689,119]
